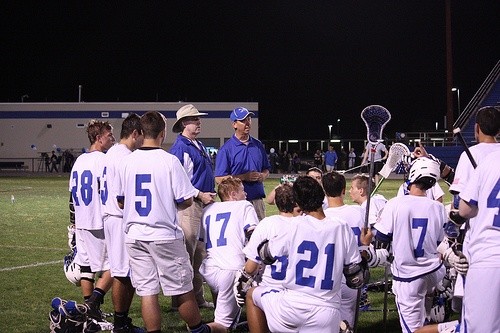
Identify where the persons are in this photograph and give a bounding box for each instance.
[373,106,500,333]
[67,119,116,323]
[116,111,214,333]
[247,174,372,333]
[169,104,216,308]
[266,132,388,180]
[100,113,144,333]
[243,184,298,288]
[306,167,388,333]
[213,108,270,221]
[190,177,259,333]
[46,150,74,172]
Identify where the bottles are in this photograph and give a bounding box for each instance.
[280,177,297,184]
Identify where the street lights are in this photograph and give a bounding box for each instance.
[328,125,333,150]
[78,85,83,103]
[451,87,462,118]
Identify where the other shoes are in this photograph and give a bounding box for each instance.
[82,297,102,322]
[113,314,140,333]
[198,301,214,308]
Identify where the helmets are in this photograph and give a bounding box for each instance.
[64,247,81,288]
[408,157,440,184]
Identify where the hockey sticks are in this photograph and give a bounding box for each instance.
[354,104,392,333]
[332,158,383,175]
[369,143,411,198]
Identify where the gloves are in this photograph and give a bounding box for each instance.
[364,245,393,268]
[444,212,467,239]
[233,273,254,309]
[443,249,469,274]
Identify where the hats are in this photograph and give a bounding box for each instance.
[171,104,208,133]
[230,107,255,122]
